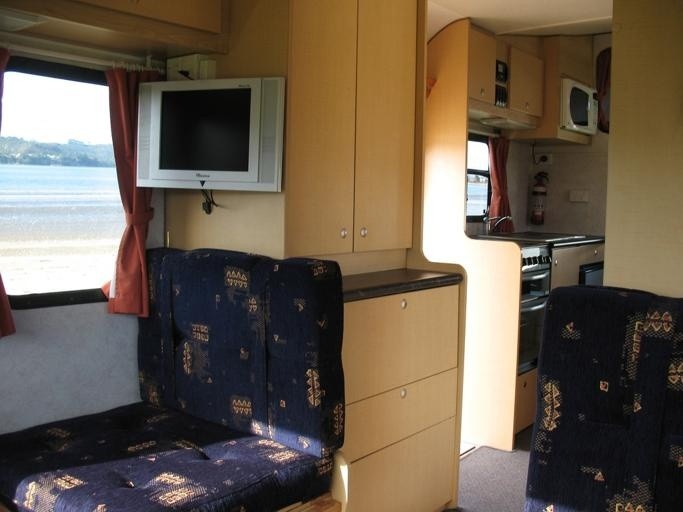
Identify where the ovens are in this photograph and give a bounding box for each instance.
[517,268,550,376]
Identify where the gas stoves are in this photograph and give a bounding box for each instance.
[468,239,552,272]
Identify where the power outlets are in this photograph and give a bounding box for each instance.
[535,152,553,165]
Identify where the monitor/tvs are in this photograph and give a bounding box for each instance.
[136,77,284,191]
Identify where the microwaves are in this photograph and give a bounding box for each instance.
[559,77,599,136]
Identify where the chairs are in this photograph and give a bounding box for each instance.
[1,243,346,511]
[522,282,682,511]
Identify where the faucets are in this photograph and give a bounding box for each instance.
[482,210,513,235]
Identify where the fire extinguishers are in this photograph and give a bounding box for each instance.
[531,172,549,225]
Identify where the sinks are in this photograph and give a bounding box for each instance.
[477,230,586,243]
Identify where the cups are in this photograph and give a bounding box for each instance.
[200,60,216,81]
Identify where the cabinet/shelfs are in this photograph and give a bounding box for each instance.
[504,30,596,148]
[161,1,418,278]
[458,371,535,436]
[426,17,543,129]
[547,242,605,287]
[327,265,462,512]
